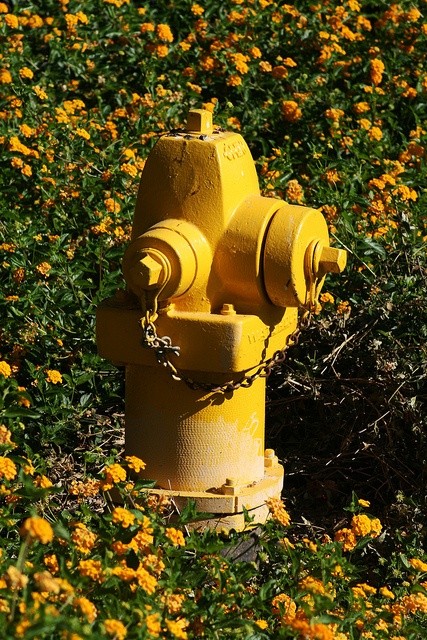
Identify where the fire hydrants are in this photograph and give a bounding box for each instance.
[94,108,348,534]
